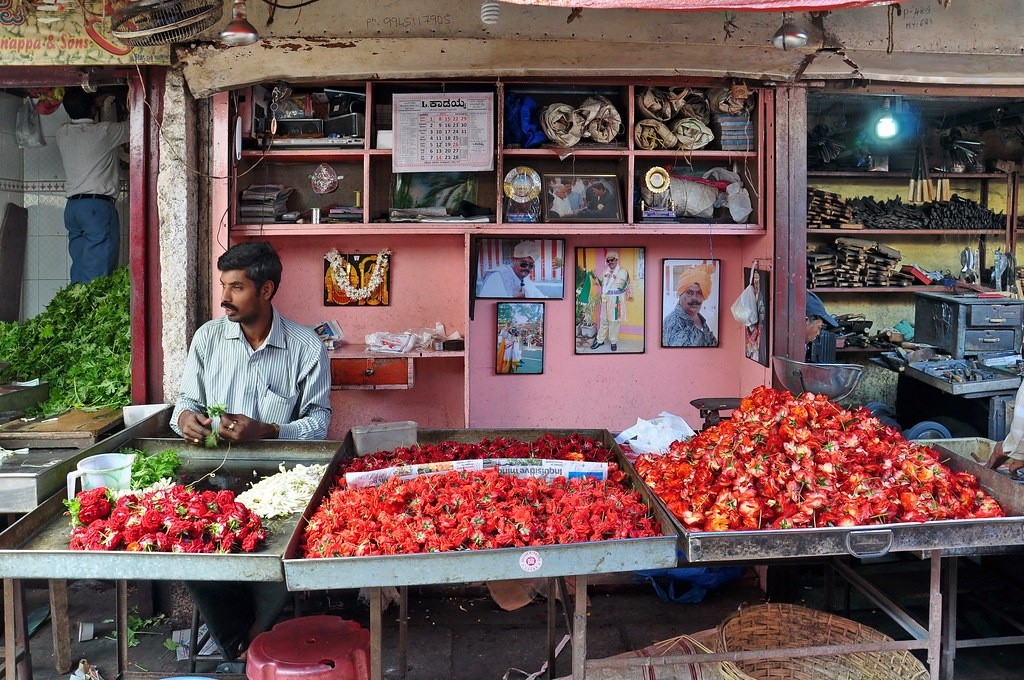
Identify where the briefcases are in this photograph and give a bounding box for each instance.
[806,329,836,364]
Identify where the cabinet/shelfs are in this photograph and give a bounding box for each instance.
[917,288,1024,365]
[1011,172,1024,276]
[212,66,769,231]
[805,169,1011,358]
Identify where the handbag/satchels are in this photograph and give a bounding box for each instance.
[730,260,758,326]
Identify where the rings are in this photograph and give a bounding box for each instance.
[228,421,237,431]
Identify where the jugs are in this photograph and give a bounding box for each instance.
[66,452,139,503]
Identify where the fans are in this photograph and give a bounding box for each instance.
[110,1,224,46]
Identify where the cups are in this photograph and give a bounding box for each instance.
[78,622,95,643]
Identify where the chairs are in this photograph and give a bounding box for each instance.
[188,594,300,672]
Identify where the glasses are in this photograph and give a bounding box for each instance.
[606,257,615,262]
[517,260,535,269]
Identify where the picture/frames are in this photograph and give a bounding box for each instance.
[495,302,545,374]
[661,259,722,348]
[540,175,625,224]
[471,240,567,300]
[575,246,649,354]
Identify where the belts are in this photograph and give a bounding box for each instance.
[68,193,116,204]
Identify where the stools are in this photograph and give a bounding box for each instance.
[245,614,371,680]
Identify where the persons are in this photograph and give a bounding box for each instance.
[169,242,331,660]
[806,289,839,341]
[547,178,617,218]
[590,251,634,351]
[746,272,765,364]
[55,86,129,283]
[984,344,1023,476]
[663,261,718,346]
[497,318,522,373]
[479,240,549,298]
[525,334,532,347]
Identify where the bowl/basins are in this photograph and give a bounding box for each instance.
[770,353,864,400]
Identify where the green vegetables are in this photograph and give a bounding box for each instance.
[120,404,232,490]
[127,603,177,673]
[62,497,82,528]
[1,264,131,418]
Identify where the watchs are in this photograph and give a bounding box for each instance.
[271,423,280,439]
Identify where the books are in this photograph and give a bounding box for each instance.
[415,214,490,223]
[238,184,294,222]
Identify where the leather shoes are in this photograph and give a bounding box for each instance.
[591,341,604,349]
[611,344,617,351]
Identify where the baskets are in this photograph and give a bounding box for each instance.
[716,603,931,680]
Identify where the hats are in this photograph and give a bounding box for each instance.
[806,290,839,326]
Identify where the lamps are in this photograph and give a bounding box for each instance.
[769,9,808,51]
[220,3,259,46]
